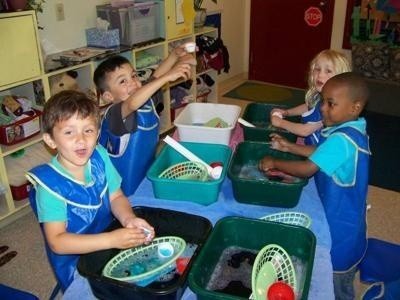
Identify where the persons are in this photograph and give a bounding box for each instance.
[258,72,375,300]
[270,48,353,146]
[7,129,15,143]
[92,41,202,197]
[12,124,25,142]
[23,89,157,293]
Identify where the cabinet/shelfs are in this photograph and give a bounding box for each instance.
[0,14,55,211]
[45,41,170,130]
[164,0,223,125]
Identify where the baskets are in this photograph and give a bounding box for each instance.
[154,160,210,184]
[249,242,299,300]
[100,234,188,284]
[259,210,313,231]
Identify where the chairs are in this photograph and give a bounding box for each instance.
[362,237,400,300]
[0,184,81,300]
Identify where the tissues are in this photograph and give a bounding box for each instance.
[85,17,121,49]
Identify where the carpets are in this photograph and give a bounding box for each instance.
[219,80,307,110]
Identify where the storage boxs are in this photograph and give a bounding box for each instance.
[146,140,232,206]
[188,215,317,300]
[227,141,309,209]
[76,205,212,300]
[239,101,301,145]
[173,102,241,146]
